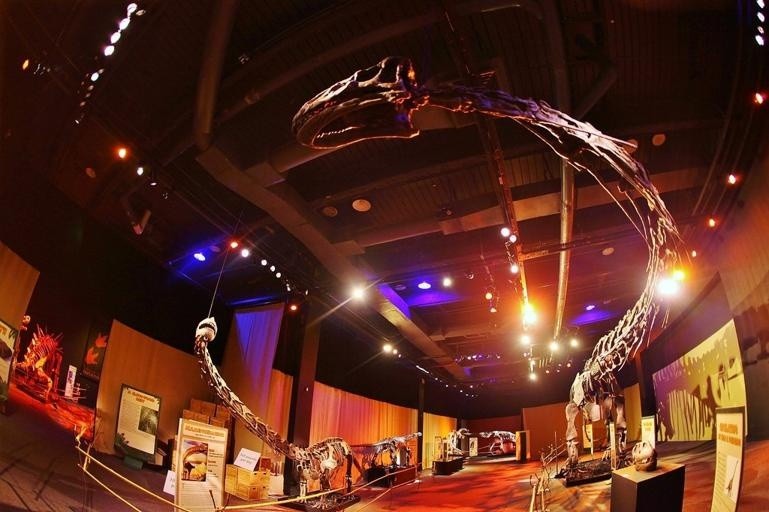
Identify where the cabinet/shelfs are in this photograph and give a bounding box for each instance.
[374,464,417,488]
[433,456,465,475]
[224,465,270,501]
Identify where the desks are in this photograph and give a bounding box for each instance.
[608,460,686,512]
